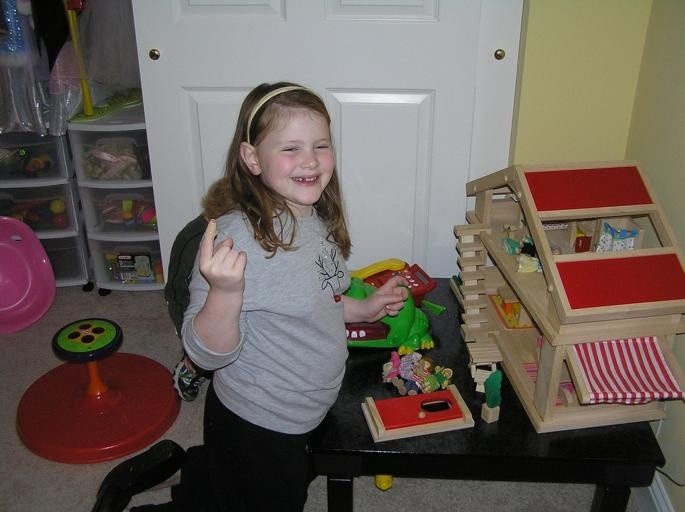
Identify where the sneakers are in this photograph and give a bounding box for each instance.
[172,361,205,401]
[97,440,186,500]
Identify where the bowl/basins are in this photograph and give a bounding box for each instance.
[1,217,56,335]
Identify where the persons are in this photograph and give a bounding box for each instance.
[181,77,410,512]
[163,176,236,402]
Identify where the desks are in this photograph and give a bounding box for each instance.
[307,273,666,512]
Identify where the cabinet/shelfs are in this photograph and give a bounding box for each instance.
[0,87,165,295]
